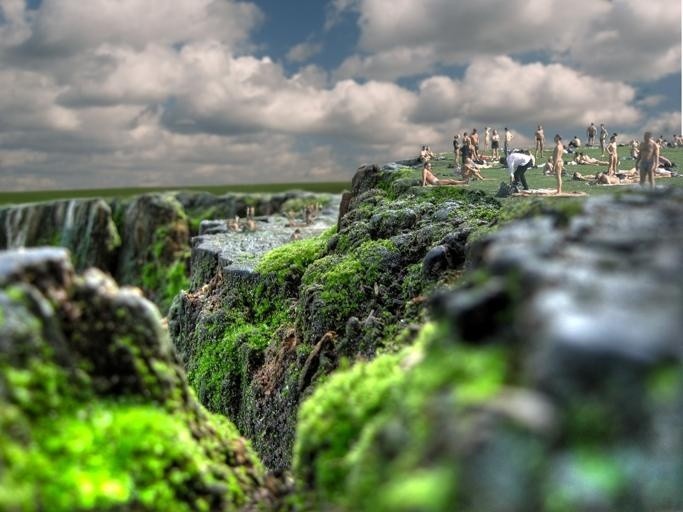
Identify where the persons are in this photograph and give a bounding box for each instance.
[225,200,319,240]
[416,121,682,195]
